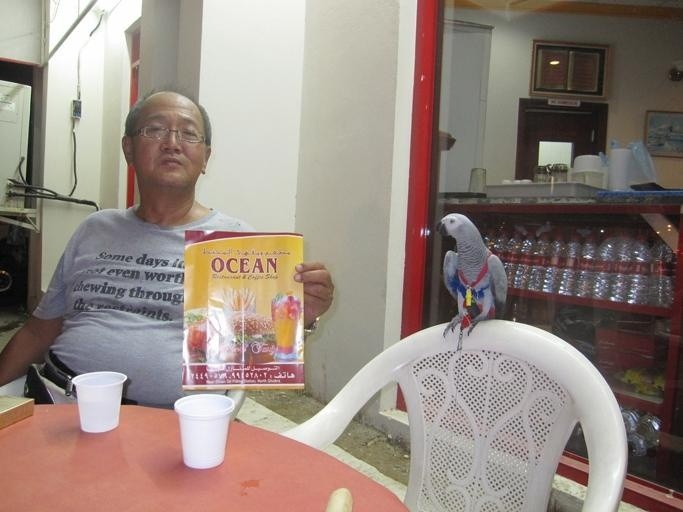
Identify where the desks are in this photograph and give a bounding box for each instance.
[0,403,410,511]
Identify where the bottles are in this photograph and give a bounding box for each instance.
[621,408,663,457]
[486,224,675,308]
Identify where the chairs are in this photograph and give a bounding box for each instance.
[0,372,248,418]
[277,318,631,511]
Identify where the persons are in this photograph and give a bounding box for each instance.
[1,90,333,408]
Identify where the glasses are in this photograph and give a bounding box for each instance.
[128,125,206,143]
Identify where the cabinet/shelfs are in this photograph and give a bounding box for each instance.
[436,194,683,484]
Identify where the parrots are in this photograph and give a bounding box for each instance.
[436,213,508,338]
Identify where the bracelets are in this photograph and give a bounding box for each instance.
[305,316,321,337]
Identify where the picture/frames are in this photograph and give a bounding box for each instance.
[643,109,683,158]
[528,39,611,101]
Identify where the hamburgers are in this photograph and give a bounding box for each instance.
[231,312,276,365]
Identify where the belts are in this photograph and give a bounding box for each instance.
[23,362,137,405]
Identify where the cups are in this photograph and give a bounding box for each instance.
[271,300,299,363]
[469,167,488,197]
[606,148,633,192]
[534,163,568,184]
[72,371,128,433]
[174,394,235,469]
